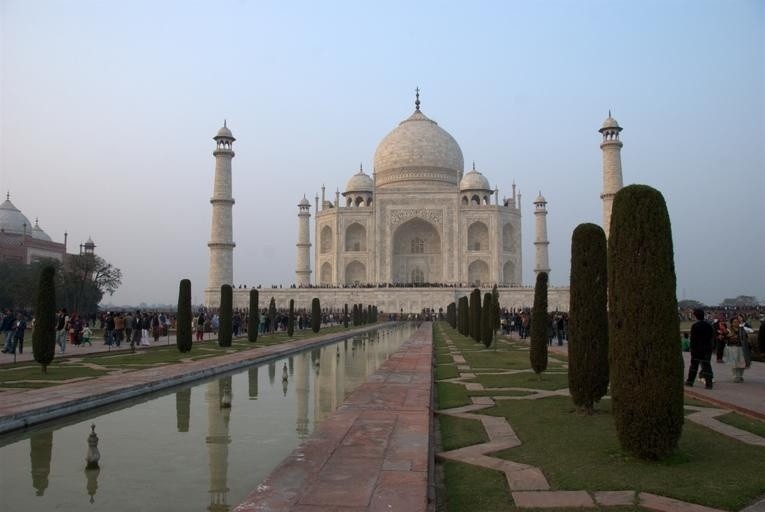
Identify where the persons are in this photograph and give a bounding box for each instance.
[0,308,171,355]
[232,282,464,288]
[388,308,436,322]
[192,306,269,340]
[678,303,765,389]
[500,308,568,346]
[274,308,345,331]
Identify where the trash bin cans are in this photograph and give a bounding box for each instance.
[432,314,435,322]
[70,331,75,343]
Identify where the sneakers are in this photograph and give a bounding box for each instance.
[57,348,67,354]
[1,347,24,355]
[683,376,713,389]
